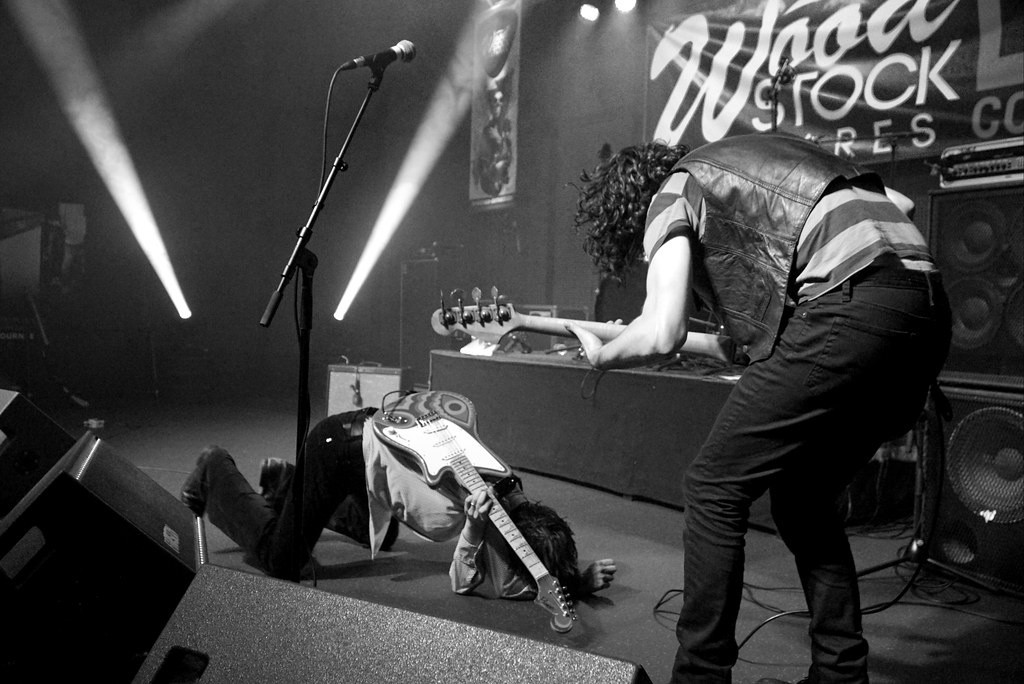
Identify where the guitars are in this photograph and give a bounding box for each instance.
[431,285,737,363]
[372,388,581,634]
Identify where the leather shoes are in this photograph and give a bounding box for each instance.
[259,459,283,507]
[180,445,219,519]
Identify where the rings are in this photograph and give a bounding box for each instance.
[470,502,477,509]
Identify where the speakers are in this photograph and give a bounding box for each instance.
[923,181,1024,598]
[398,258,478,399]
[130,565,654,684]
[514,305,588,351]
[0,389,208,684]
[324,364,400,417]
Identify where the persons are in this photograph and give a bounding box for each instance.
[560,130,959,684]
[182,388,620,598]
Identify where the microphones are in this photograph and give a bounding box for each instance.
[337,39,416,71]
[352,380,361,405]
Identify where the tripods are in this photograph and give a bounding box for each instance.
[856,393,1016,598]
